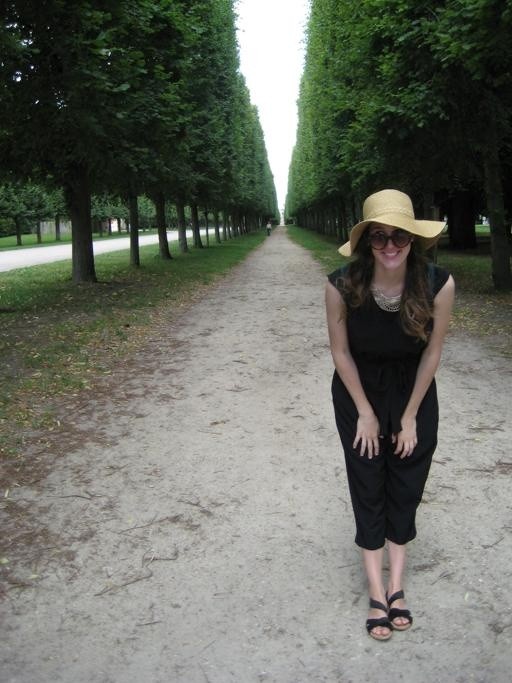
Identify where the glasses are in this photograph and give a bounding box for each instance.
[366,228,412,249]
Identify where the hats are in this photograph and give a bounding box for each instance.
[337,188,446,257]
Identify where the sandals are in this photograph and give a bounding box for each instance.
[365,588,414,641]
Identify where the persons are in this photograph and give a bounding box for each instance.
[324,187,456,641]
[266,221,271,236]
